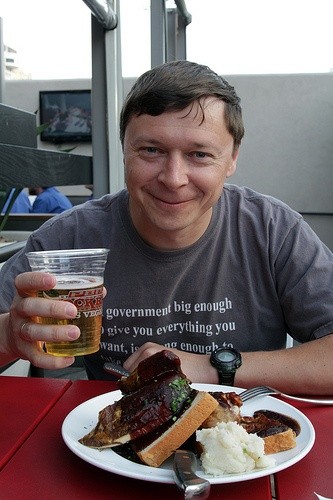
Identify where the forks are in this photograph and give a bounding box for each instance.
[237,386,333,405]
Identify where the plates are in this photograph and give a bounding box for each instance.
[62,383,316,484]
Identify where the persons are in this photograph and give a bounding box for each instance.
[0,61,333,394]
[28,186,73,214]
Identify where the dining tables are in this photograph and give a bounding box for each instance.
[1,376,333,500]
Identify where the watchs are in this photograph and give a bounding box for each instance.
[210,346,242,387]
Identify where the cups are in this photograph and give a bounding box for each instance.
[25,248,111,356]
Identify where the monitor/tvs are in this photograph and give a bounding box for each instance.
[39,89,92,144]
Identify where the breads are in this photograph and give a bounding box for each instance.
[264,428,296,454]
[129,389,219,467]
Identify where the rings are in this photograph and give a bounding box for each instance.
[20,323,26,341]
